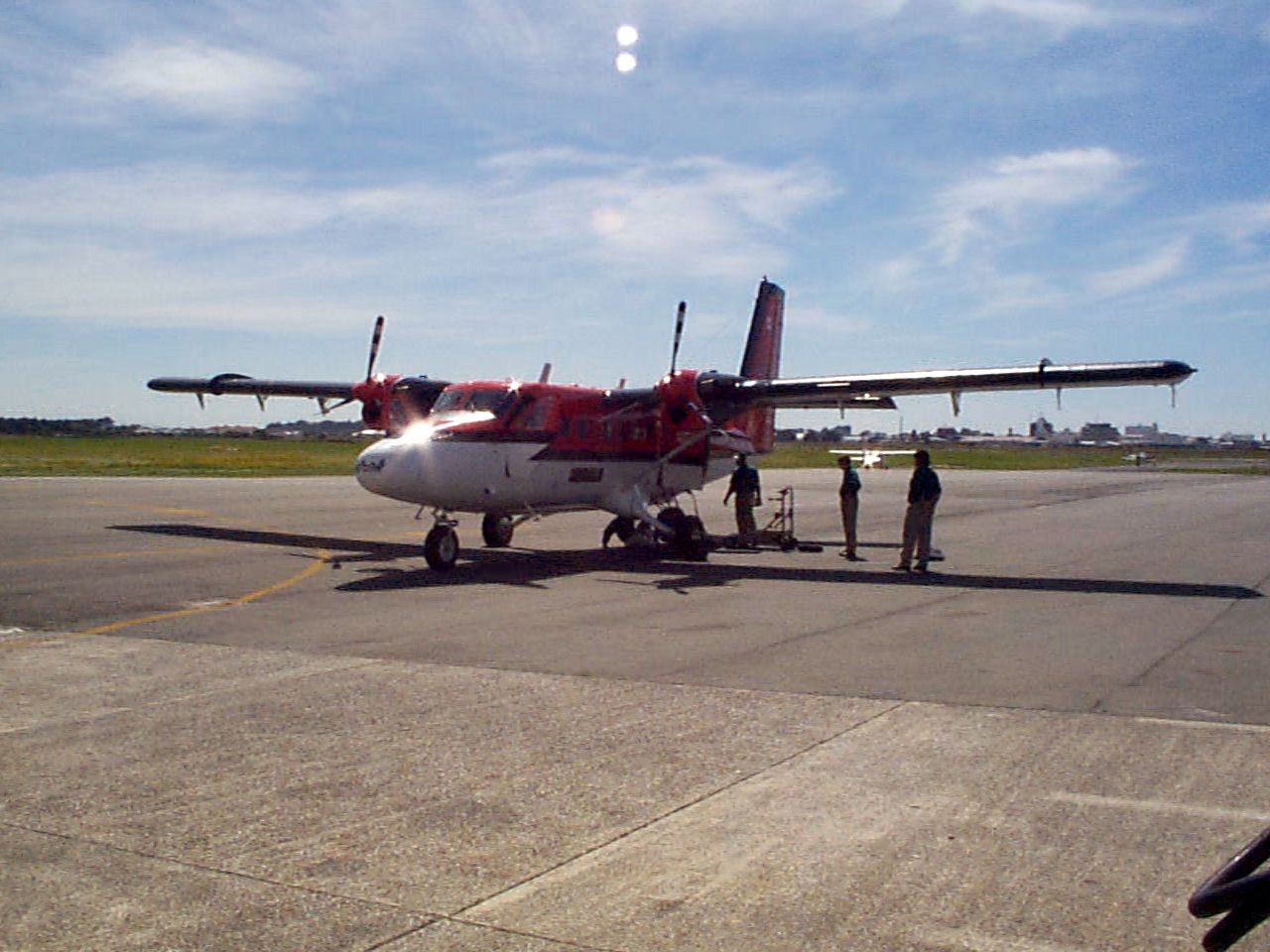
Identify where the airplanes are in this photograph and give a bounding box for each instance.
[146,277,1201,577]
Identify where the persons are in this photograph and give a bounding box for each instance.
[837,455,861,560]
[892,451,942,572]
[723,453,762,548]
[603,508,705,553]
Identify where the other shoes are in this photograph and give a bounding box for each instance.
[912,565,925,573]
[891,563,911,572]
